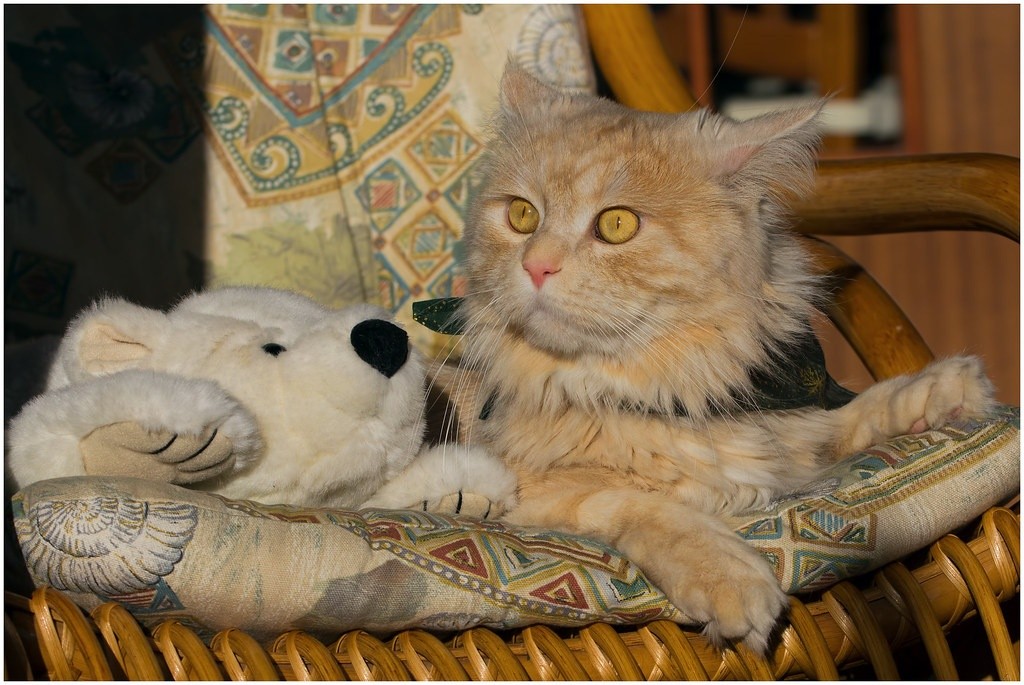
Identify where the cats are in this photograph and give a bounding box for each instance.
[411,53,995,654]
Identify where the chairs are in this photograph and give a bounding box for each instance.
[3,3,1022,682]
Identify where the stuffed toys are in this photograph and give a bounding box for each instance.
[8,284,520,508]
